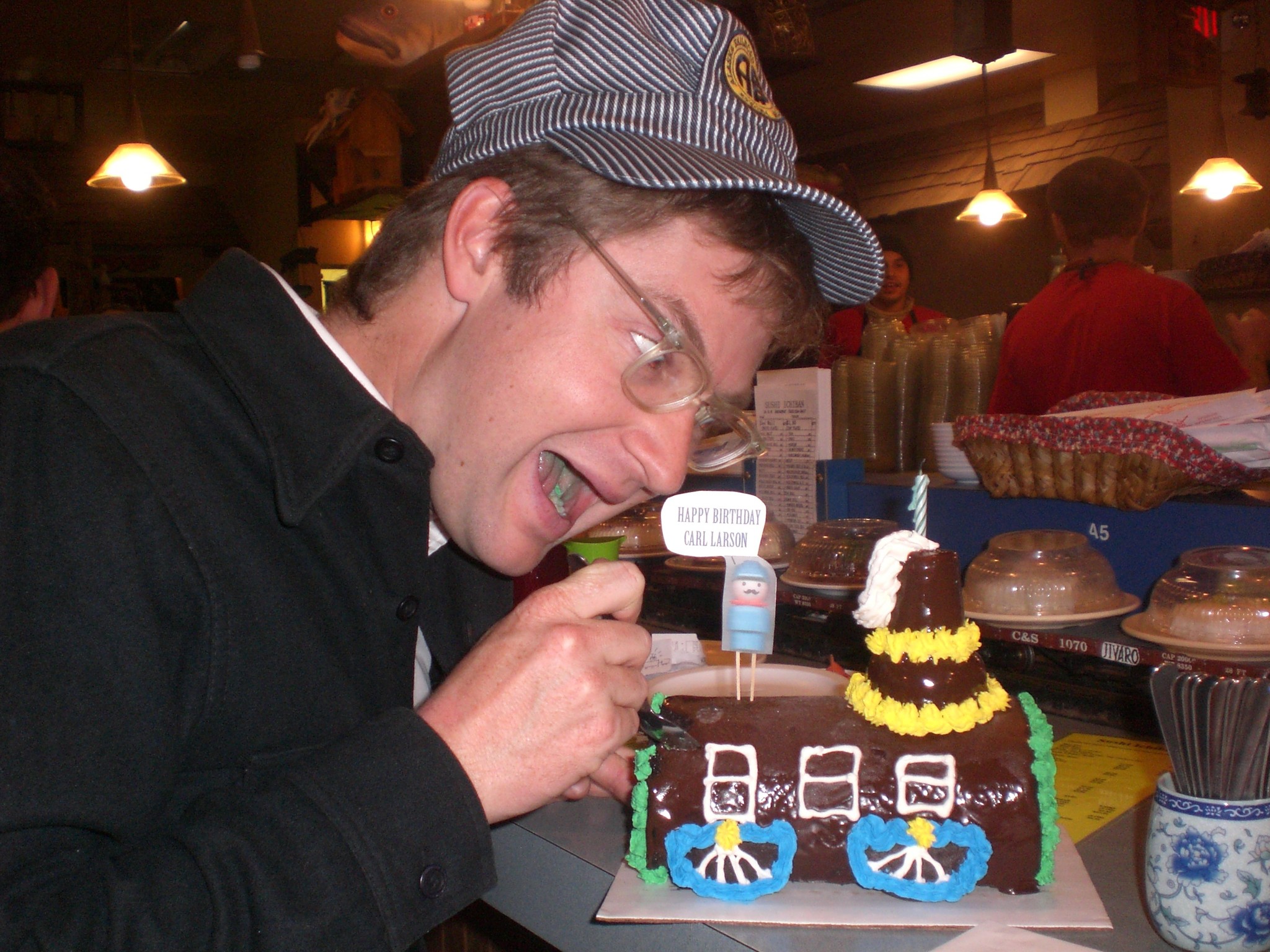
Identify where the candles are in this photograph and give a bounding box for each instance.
[907,458,930,538]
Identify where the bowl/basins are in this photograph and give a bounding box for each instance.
[831,315,1006,471]
[578,503,1270,662]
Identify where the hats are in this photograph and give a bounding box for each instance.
[427,0,884,304]
[877,234,913,280]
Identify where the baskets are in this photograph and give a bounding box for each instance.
[953,391,1270,512]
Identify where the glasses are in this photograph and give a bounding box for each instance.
[522,196,768,476]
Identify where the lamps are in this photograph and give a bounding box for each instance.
[1179,82,1263,201]
[86,2,186,190]
[956,65,1027,225]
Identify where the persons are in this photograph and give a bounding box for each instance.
[820,234,945,370]
[986,156,1262,415]
[0,0,885,952]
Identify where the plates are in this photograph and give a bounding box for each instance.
[964,594,1142,631]
[664,556,790,574]
[1121,615,1270,664]
[930,422,981,484]
[618,550,676,559]
[778,574,864,598]
[646,664,850,716]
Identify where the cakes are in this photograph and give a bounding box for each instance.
[626,528,1059,903]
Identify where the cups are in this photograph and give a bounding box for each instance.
[1142,772,1270,952]
[563,535,626,564]
